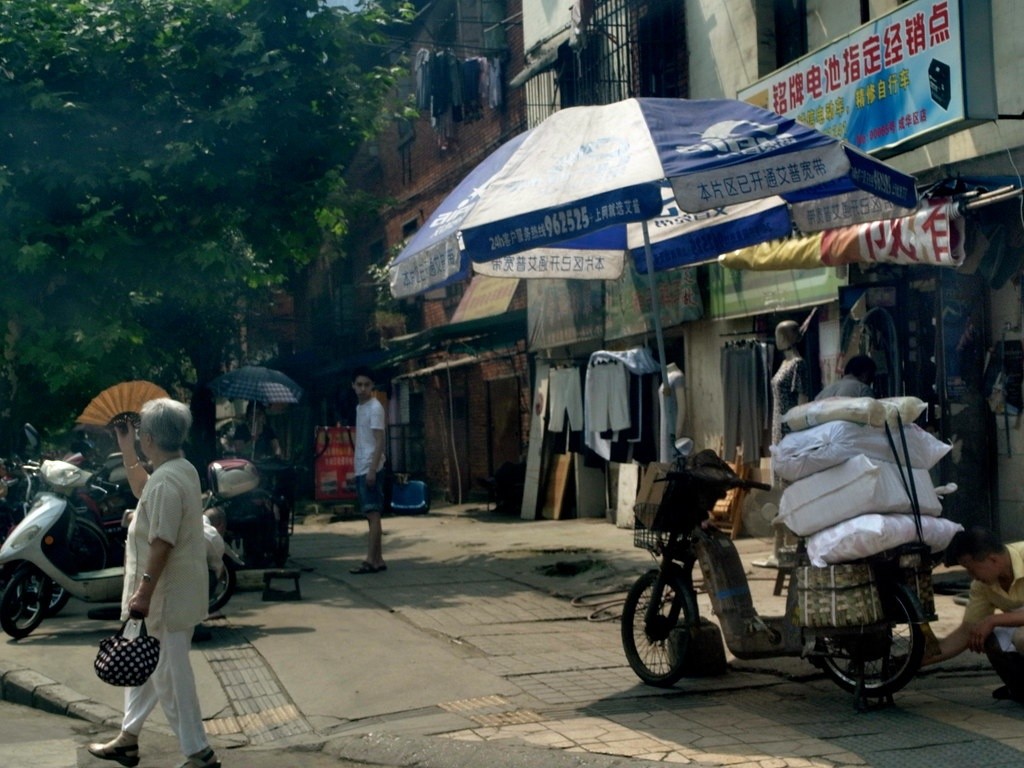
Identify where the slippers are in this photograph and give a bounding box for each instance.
[350,561,386,575]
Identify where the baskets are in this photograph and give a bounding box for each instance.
[632,503,671,554]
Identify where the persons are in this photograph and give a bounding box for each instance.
[219,400,283,461]
[87,398,222,768]
[349,365,388,575]
[769,320,810,559]
[656,362,688,464]
[884,528,1024,709]
[813,355,881,399]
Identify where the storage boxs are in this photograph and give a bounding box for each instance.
[792,560,885,627]
[887,546,936,622]
[632,460,671,529]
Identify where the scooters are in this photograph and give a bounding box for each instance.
[620,437,936,712]
[0,421,262,638]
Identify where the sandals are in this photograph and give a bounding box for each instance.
[180,750,221,768]
[87,741,140,767]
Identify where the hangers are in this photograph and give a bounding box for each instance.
[546,356,619,370]
[400,38,501,61]
[723,330,774,349]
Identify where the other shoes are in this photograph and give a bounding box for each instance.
[993,685,1024,701]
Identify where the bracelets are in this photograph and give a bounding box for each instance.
[124,456,139,469]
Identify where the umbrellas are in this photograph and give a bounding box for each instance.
[387,97,918,432]
[203,365,305,461]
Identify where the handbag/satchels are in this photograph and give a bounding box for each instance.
[94,614,161,688]
